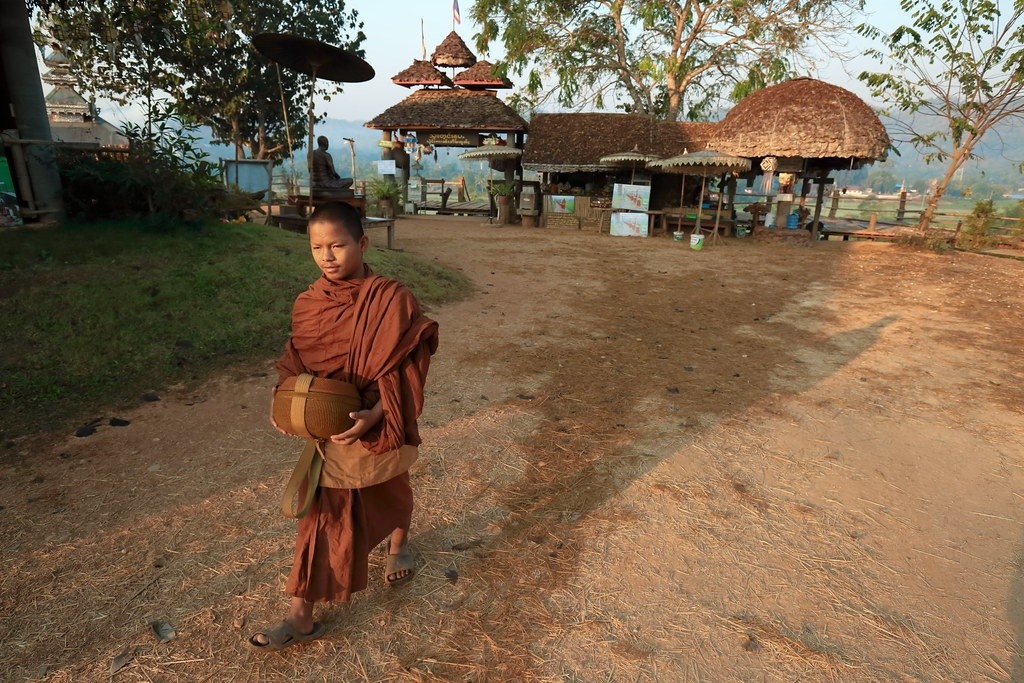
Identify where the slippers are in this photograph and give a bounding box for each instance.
[247,620,326,651]
[384,538,415,586]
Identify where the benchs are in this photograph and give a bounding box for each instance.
[659,219,734,237]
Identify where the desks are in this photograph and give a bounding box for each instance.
[264,213,395,250]
[288,194,366,219]
[645,210,666,237]
[593,207,618,234]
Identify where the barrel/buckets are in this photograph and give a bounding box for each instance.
[674,231,684,241]
[765,213,776,228]
[690,234,704,251]
[786,214,799,229]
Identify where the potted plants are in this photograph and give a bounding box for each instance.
[366,175,412,207]
[485,180,521,204]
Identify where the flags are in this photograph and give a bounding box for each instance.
[453,0,460,25]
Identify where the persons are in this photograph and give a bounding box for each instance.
[692,176,707,204]
[246,198,439,655]
[310,137,353,189]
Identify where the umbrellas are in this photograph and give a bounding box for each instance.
[599,144,751,234]
[458,144,524,224]
[251,31,376,198]
